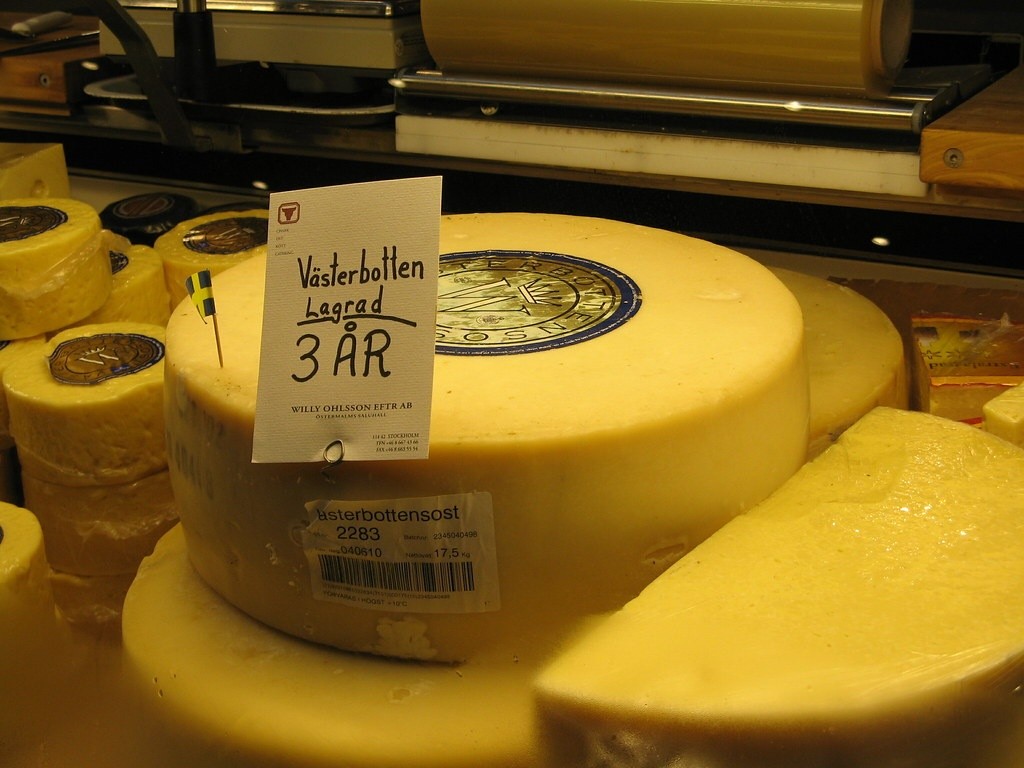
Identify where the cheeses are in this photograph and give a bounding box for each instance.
[1,187,1023,768]
